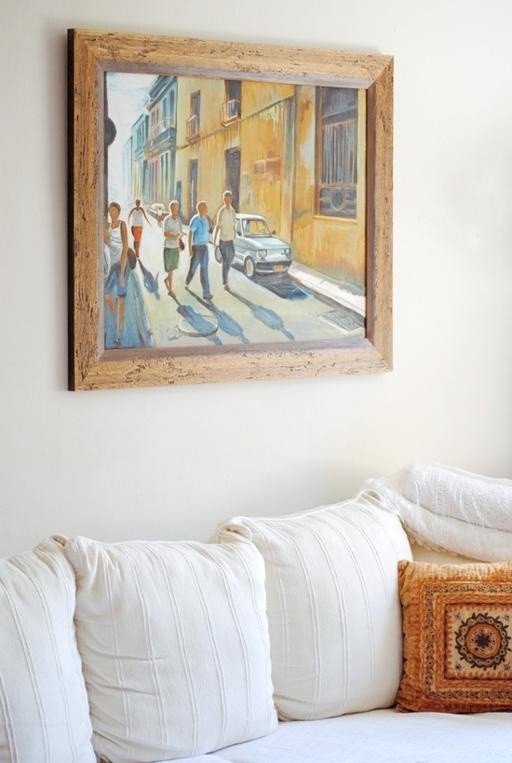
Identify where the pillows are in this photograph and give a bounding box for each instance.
[0,533,103,763]
[396,559,512,712]
[232,490,414,729]
[65,522,283,763]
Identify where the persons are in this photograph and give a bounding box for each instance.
[163,201,183,297]
[185,202,213,299]
[213,191,237,290]
[104,203,128,346]
[128,200,150,257]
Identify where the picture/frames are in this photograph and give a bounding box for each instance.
[66,27,393,393]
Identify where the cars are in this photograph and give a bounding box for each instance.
[215,213,292,282]
[148,199,170,221]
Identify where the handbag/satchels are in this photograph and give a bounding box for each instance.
[127,247,137,270]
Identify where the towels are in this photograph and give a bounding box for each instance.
[366,479,512,568]
[408,462,512,533]
